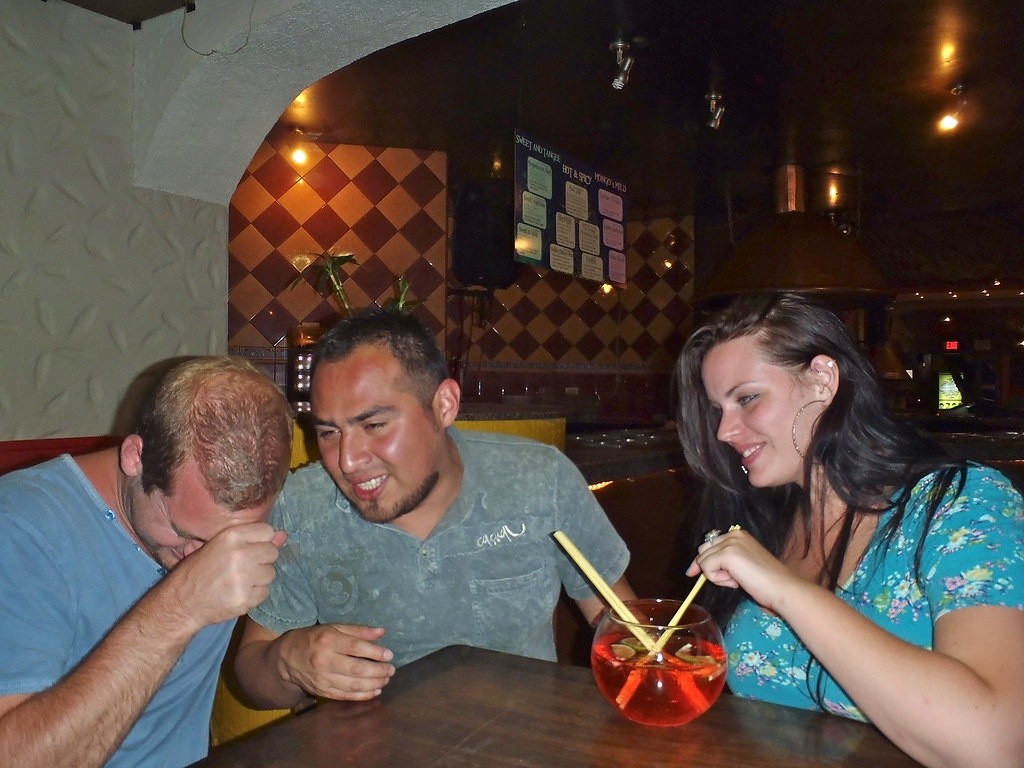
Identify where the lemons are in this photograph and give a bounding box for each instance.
[611,636,714,664]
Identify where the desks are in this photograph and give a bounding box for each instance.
[181,643,926,768]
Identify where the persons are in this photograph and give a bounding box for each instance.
[678,286,1024,768]
[220,310,653,707]
[0,353,294,768]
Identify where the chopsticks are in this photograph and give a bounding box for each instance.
[617,524,741,710]
[553,531,708,710]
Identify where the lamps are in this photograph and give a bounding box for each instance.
[608,40,635,90]
[706,93,725,129]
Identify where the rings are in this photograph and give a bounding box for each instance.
[705,529,721,545]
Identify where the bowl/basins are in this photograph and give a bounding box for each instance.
[590,599,730,727]
